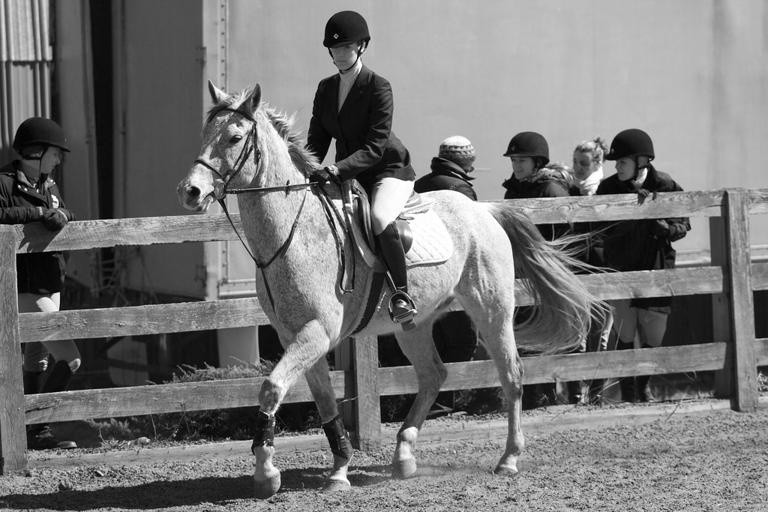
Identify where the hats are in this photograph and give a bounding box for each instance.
[439,136,476,172]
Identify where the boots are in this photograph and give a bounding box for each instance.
[22,359,77,449]
[637,343,657,403]
[375,221,414,324]
[616,336,635,404]
[589,378,603,405]
[567,345,584,404]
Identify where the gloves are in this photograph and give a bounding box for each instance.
[629,187,650,197]
[309,166,336,187]
[43,209,71,232]
[647,219,669,238]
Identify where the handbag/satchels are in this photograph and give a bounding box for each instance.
[635,242,676,307]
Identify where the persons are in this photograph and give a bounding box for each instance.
[413,133,479,420]
[567,137,614,406]
[592,126,691,402]
[301,10,419,333]
[0,117,83,449]
[500,129,578,410]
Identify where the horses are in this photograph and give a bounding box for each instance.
[175,79,628,499]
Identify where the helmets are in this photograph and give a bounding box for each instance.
[323,11,370,48]
[13,118,71,153]
[603,129,655,160]
[503,133,550,165]
